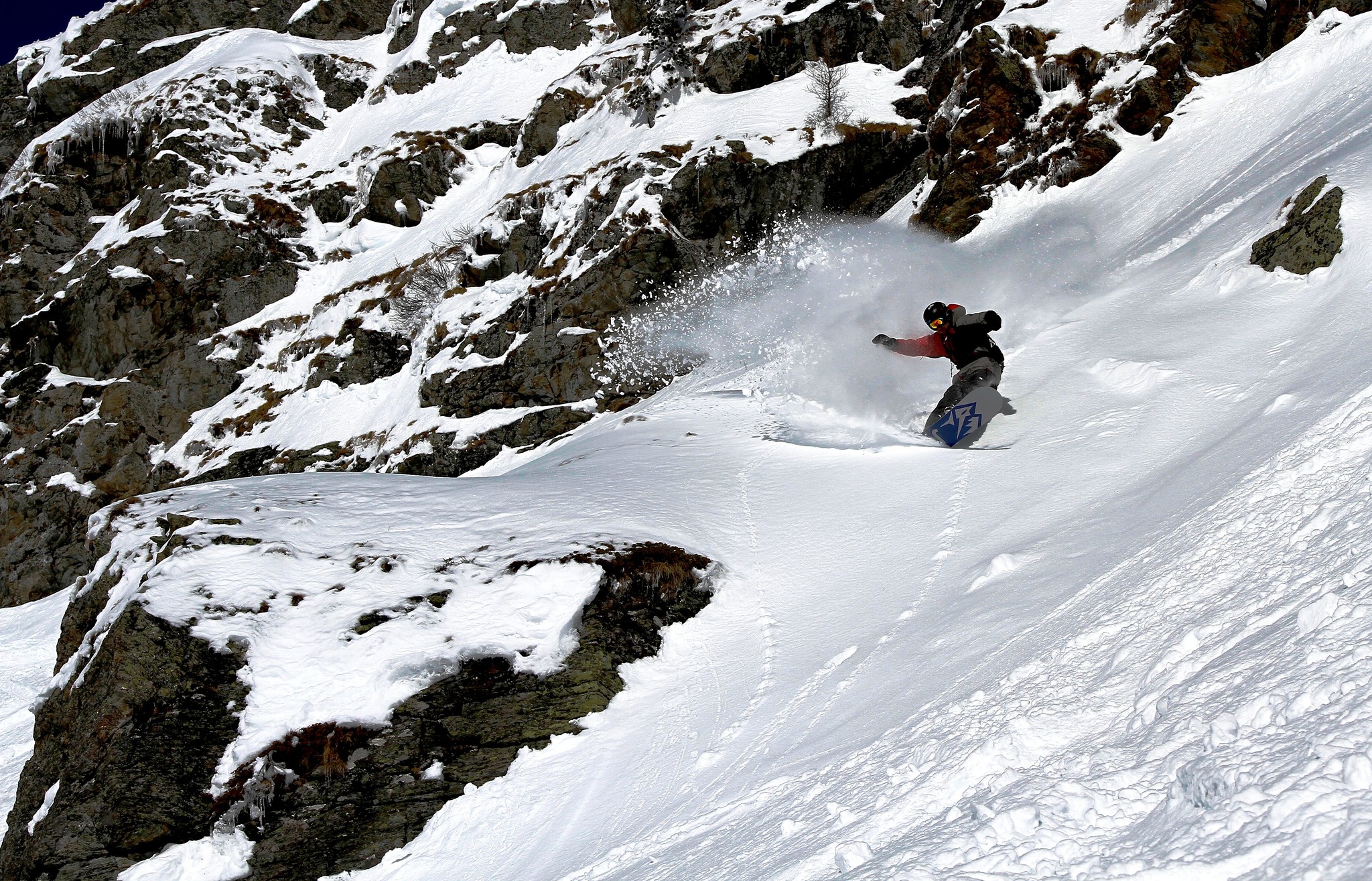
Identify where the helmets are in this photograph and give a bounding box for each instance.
[923,302,948,325]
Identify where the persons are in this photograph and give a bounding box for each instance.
[872,302,1005,434]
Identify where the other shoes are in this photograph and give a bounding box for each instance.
[944,406,953,415]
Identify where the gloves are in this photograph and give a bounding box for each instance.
[985,310,1002,331]
[873,334,896,348]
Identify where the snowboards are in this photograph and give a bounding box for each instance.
[921,385,1003,447]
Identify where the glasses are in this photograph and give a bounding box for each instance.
[929,317,946,330]
